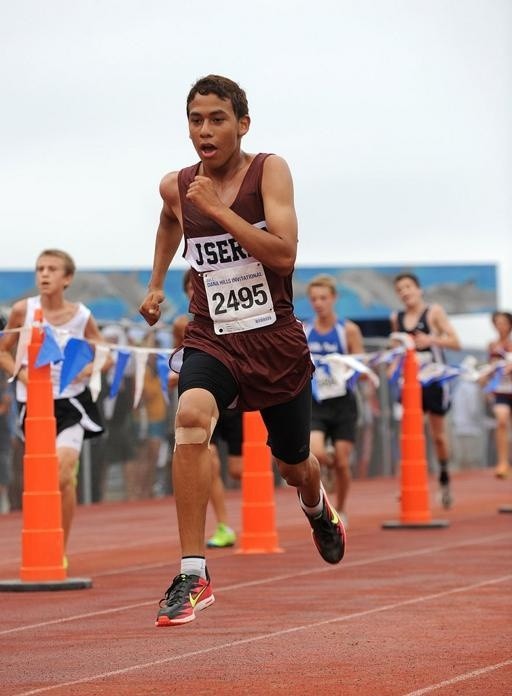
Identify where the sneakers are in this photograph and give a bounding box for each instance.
[206,522,235,546]
[494,462,509,479]
[299,480,346,564]
[154,566,215,627]
[441,486,452,509]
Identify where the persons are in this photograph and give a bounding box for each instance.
[135,71,347,630]
[387,270,463,511]
[1,247,116,576]
[299,273,368,532]
[167,268,246,548]
[0,305,511,515]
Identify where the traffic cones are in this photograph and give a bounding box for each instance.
[222,406,289,560]
[4,302,100,592]
[380,351,452,532]
